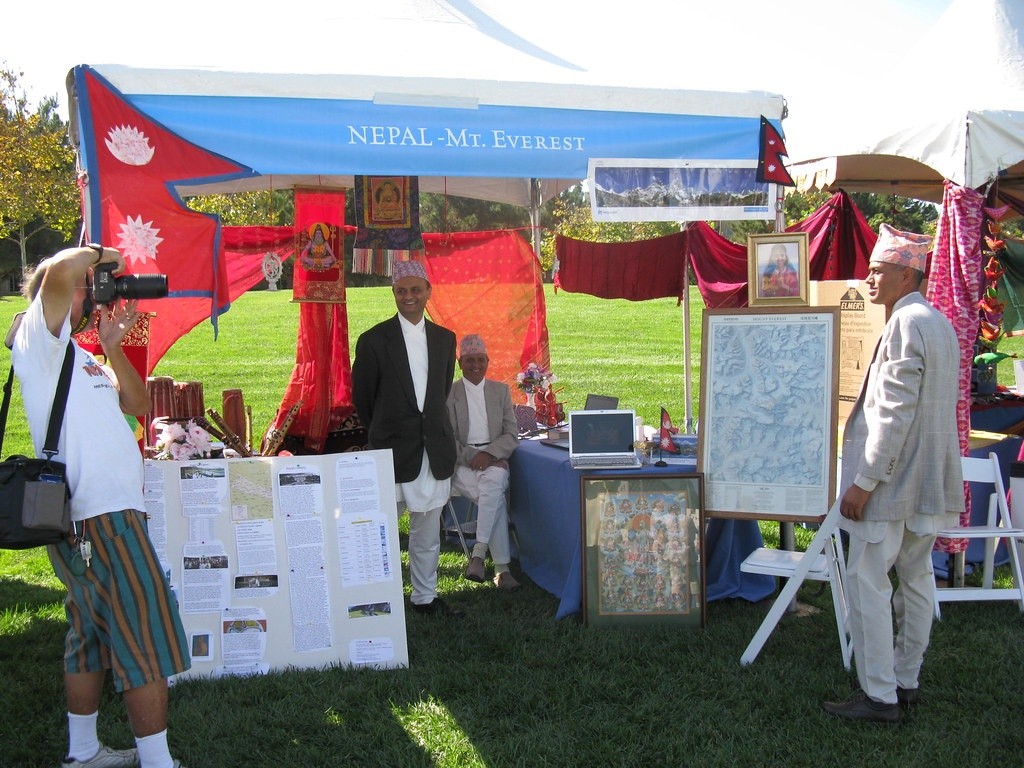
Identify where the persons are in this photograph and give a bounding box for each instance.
[350,261,466,619]
[11,244,193,768]
[822,222,966,723]
[444,334,523,592]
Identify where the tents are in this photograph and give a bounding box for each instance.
[64,65,798,614]
[768,0,1023,606]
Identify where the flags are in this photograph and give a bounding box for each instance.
[660,428,677,453]
[661,409,679,435]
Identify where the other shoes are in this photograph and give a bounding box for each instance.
[494,572,522,591]
[465,557,485,584]
[410,597,465,618]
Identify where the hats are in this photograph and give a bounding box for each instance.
[392,258,430,287]
[869,222,932,274]
[460,334,486,356]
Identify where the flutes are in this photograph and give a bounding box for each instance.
[190,401,304,457]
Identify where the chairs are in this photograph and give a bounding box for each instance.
[929,452,1024,621]
[740,495,855,671]
[444,483,521,570]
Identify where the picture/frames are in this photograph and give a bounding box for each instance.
[748,232,811,308]
[579,473,707,630]
[698,306,841,524]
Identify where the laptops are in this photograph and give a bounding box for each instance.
[568,409,643,470]
[539,394,619,448]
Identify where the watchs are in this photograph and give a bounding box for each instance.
[88,243,103,264]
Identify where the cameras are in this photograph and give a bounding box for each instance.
[94,261,169,304]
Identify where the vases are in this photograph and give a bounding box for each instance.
[525,392,537,413]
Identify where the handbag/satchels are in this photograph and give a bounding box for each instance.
[0,454,71,550]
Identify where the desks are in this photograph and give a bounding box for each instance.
[445,422,777,618]
[927,399,1024,579]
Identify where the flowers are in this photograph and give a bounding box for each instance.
[514,363,557,395]
[151,417,212,460]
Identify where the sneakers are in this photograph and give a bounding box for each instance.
[822,678,918,722]
[61,740,140,768]
[172,759,185,768]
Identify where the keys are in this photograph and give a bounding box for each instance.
[69,539,92,577]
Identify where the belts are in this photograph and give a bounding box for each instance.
[468,442,490,448]
